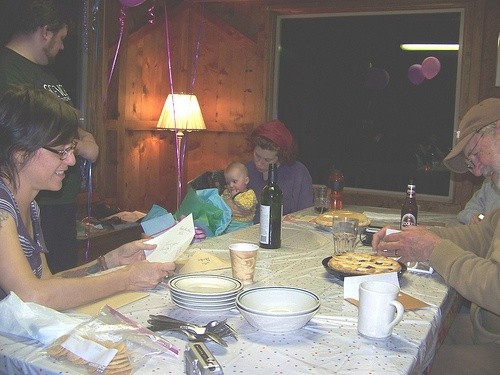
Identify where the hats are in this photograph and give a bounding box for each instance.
[253,119,293,151]
[443,98,500,174]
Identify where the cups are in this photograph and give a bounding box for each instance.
[313,184,330,212]
[364,227,381,246]
[357,281,405,341]
[229,243,259,285]
[332,216,358,255]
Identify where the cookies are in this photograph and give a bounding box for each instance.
[49,334,133,375]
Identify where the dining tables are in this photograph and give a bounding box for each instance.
[0,205,463,375]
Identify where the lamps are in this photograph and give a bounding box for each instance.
[156,91,207,151]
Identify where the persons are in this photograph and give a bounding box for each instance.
[457,176,500,225]
[245,120,313,226]
[0,82,188,312]
[220,161,257,235]
[371,96,500,375]
[0,0,99,274]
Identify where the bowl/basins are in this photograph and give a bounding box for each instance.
[235,286,321,333]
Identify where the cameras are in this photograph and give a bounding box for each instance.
[184,341,224,375]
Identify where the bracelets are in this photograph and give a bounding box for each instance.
[98,255,108,270]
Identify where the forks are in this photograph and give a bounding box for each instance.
[148,314,239,341]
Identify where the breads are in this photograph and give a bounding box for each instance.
[315,210,372,228]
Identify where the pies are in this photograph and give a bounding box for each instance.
[328,251,402,275]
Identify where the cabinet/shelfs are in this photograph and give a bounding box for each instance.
[75,222,143,265]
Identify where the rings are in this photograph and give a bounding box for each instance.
[395,249,398,257]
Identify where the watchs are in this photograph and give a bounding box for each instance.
[477,213,484,221]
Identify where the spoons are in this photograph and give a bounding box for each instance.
[204,332,228,348]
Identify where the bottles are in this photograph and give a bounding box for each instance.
[327,164,343,210]
[400,179,418,231]
[259,162,284,249]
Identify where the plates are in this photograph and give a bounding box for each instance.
[315,221,370,232]
[168,274,244,313]
[322,255,407,281]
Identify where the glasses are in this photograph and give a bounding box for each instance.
[40,140,80,161]
[464,127,488,174]
